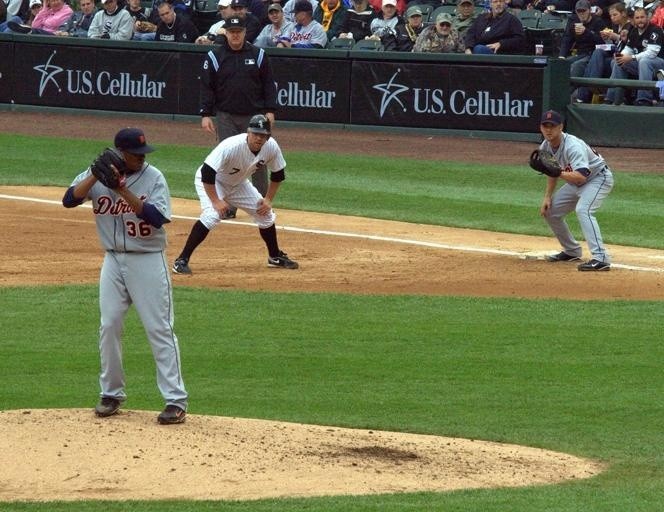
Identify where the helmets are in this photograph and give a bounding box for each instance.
[247,114,270,135]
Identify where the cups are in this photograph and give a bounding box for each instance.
[535,44,544,56]
[574,23,583,35]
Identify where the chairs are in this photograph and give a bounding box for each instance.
[139,0,569,52]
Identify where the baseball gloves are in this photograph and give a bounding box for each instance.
[91,147,126,188]
[529,149,561,177]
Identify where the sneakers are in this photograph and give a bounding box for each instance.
[171,257,193,275]
[157,405,186,424]
[544,251,579,262]
[576,258,611,272]
[94,397,121,416]
[267,250,298,270]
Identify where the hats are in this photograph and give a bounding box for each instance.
[219,16,247,29]
[574,1,589,13]
[289,1,312,13]
[381,0,397,8]
[456,1,474,7]
[28,0,42,9]
[406,6,423,18]
[114,128,153,155]
[435,13,453,25]
[267,4,282,14]
[540,110,562,126]
[214,0,248,10]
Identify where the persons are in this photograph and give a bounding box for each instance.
[173,112,299,275]
[534,109,614,273]
[62,128,190,424]
[0,1,663,105]
[200,17,277,219]
